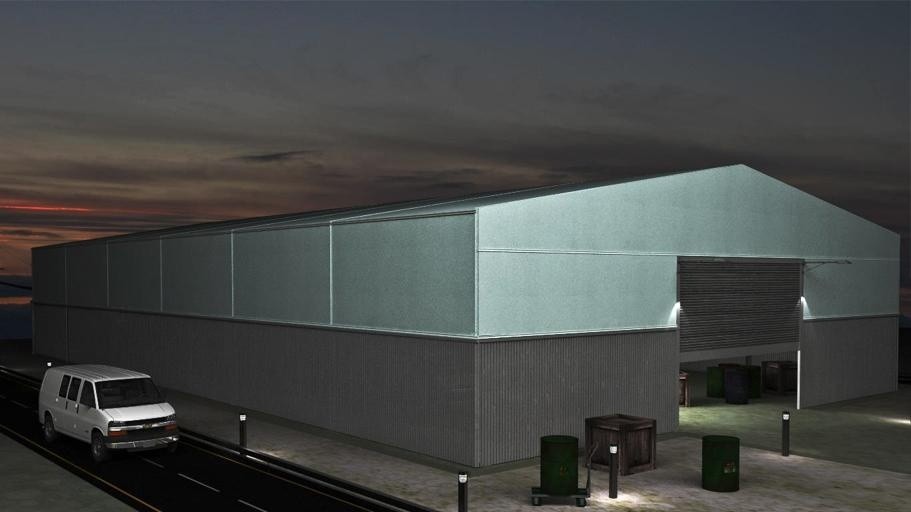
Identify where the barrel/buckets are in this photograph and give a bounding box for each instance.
[702,435,739,492]
[707,359,787,404]
[541,435,579,492]
